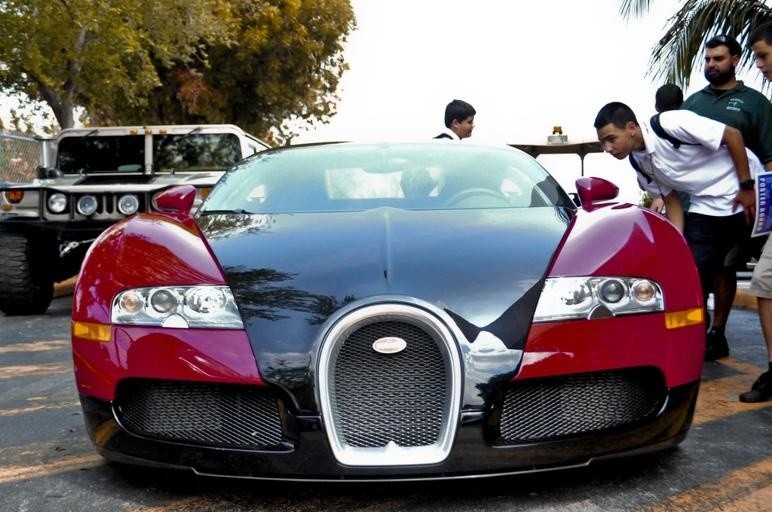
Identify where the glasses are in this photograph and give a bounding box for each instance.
[704,35,730,47]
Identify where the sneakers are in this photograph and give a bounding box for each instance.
[702,333,730,361]
[739,373,771,404]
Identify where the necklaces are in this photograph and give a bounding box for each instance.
[638,155,665,199]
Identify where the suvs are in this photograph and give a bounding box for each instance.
[0,124,270,317]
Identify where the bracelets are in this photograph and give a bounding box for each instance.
[738,178,757,192]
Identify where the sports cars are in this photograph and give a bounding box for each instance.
[67,135,711,493]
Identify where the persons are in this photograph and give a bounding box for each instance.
[591,101,770,365]
[737,23,770,400]
[428,98,475,141]
[649,82,683,216]
[683,33,771,359]
